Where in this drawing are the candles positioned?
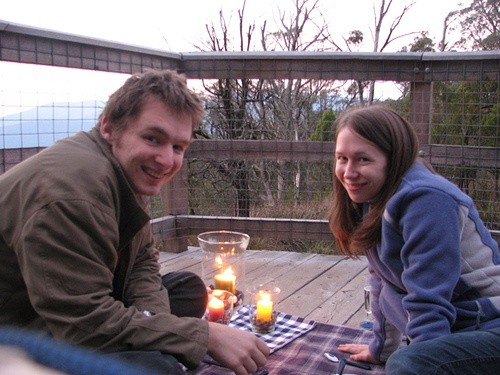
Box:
[214,265,237,295]
[255,291,274,322]
[206,296,228,323]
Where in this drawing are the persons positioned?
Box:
[328,105,500,375]
[0,68,271,375]
